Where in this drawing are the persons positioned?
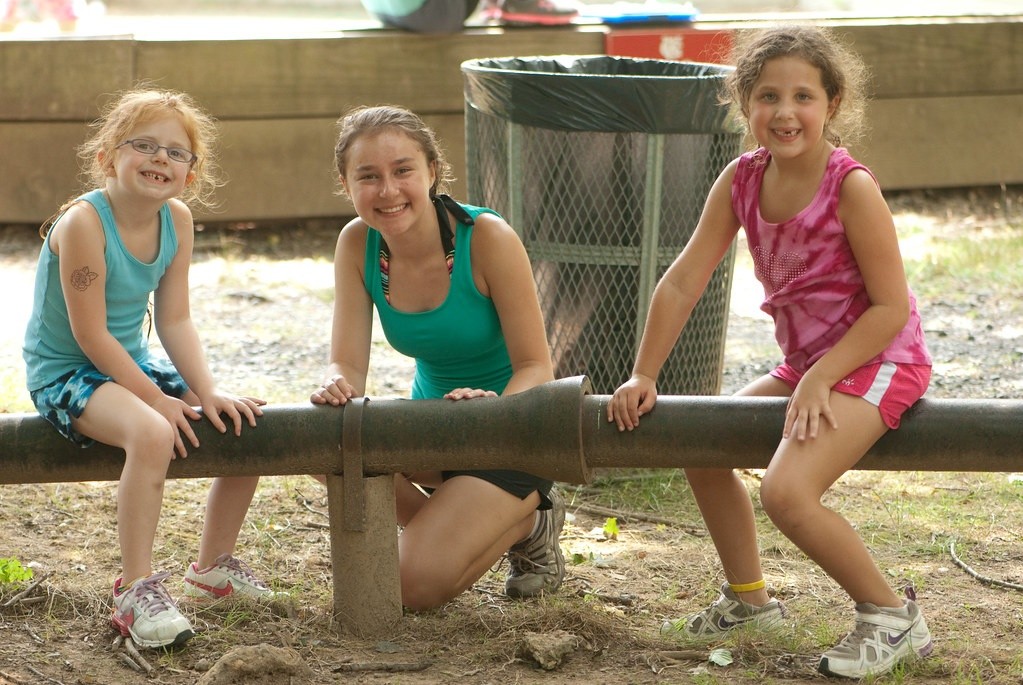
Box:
[605,22,937,682]
[306,103,570,614]
[20,84,293,653]
[352,0,585,39]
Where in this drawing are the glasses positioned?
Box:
[115,138,198,168]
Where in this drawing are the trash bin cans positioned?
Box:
[459,55,748,489]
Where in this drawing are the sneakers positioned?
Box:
[818,586,935,681]
[505,487,566,597]
[184,561,290,603]
[660,581,791,639]
[111,577,196,650]
